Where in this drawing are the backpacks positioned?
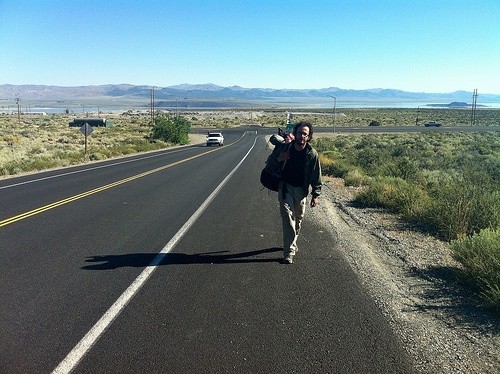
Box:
[260,140,292,193]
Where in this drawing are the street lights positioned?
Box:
[175,97,179,121]
[326,95,336,133]
[152,85,158,124]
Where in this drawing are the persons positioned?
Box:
[267,120,323,264]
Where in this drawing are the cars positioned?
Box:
[424,121,442,127]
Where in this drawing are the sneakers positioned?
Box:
[283,255,294,264]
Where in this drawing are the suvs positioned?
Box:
[206,133,225,147]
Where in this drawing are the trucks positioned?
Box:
[69,117,106,127]
